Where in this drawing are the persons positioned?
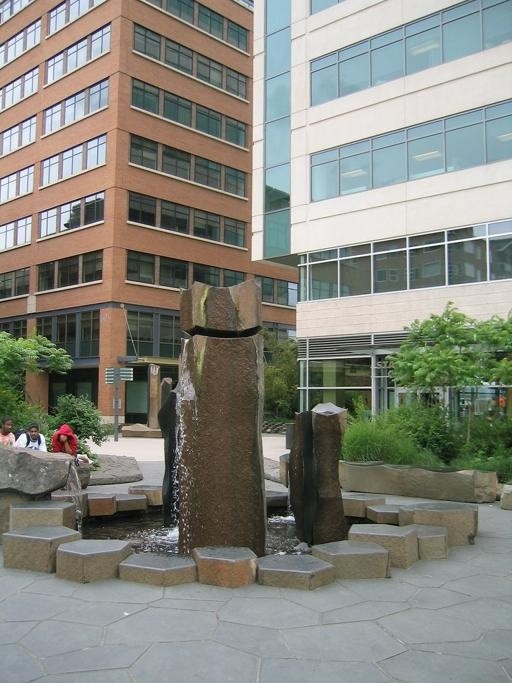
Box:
[51,422,77,455]
[15,421,47,453]
[0,415,17,450]
